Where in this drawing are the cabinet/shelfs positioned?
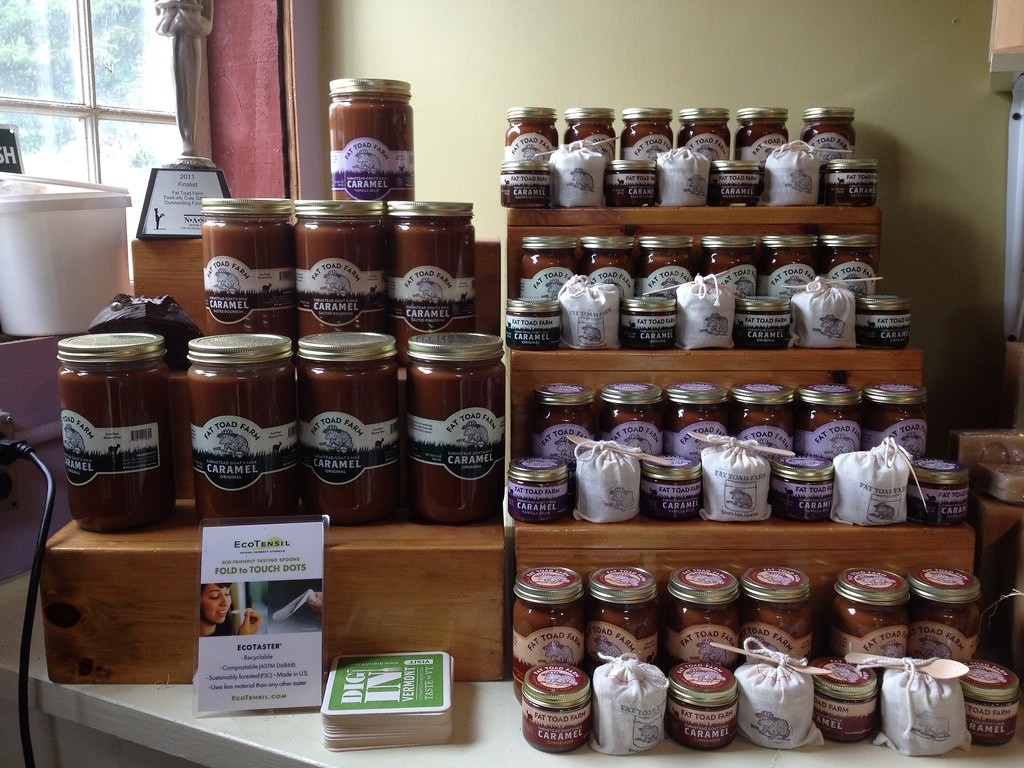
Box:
[41,237,509,681]
[501,202,980,605]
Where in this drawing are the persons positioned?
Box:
[306,591,322,622]
[200,582,262,636]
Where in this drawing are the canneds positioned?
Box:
[54,78,1022,752]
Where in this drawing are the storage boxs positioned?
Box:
[0,170,133,336]
